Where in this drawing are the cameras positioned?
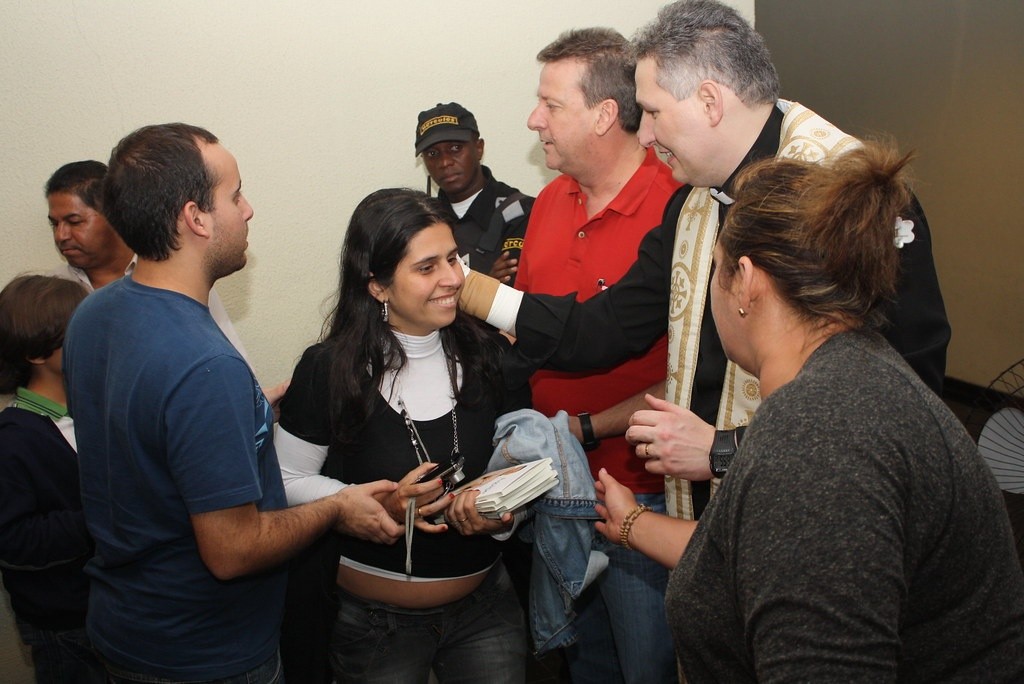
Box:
[413,452,467,488]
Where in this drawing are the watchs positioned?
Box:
[708,429,737,479]
[578,412,600,452]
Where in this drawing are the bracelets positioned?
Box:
[619,504,653,550]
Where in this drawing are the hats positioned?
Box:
[415,102,480,159]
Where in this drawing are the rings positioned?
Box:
[645,444,651,457]
[418,508,423,518]
[459,517,468,523]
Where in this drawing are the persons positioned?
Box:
[274,188,535,684]
[46,160,252,371]
[457,465,526,491]
[449,0,951,578]
[0,274,93,684]
[594,138,1024,684]
[64,123,405,684]
[492,26,684,684]
[414,102,539,410]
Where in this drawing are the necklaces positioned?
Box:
[389,360,464,490]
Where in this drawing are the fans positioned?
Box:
[964,358,1023,572]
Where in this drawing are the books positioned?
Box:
[434,458,560,525]
